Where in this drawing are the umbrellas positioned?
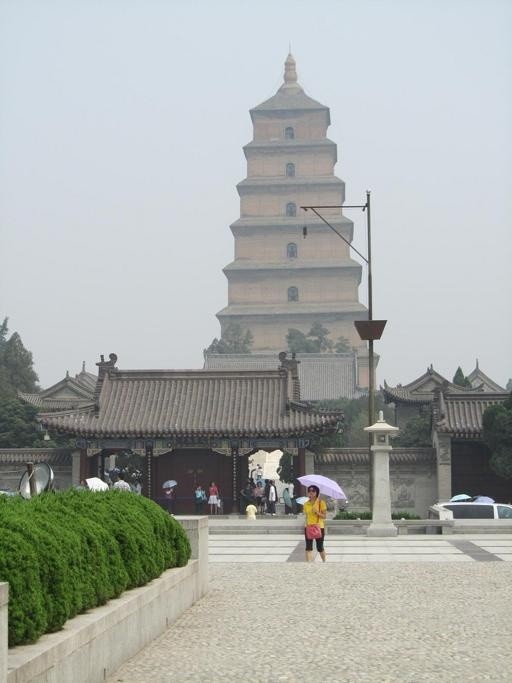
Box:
[450,492,472,500]
[295,495,311,505]
[295,473,346,499]
[473,496,494,504]
[162,479,179,488]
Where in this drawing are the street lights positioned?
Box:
[301,189,382,517]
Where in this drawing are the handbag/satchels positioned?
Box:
[305,523,323,540]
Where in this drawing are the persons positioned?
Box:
[302,484,327,561]
[195,484,207,514]
[73,464,143,499]
[239,464,298,516]
[207,480,219,514]
[166,487,177,514]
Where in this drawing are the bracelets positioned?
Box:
[314,511,318,514]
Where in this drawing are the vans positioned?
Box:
[429,499,512,519]
